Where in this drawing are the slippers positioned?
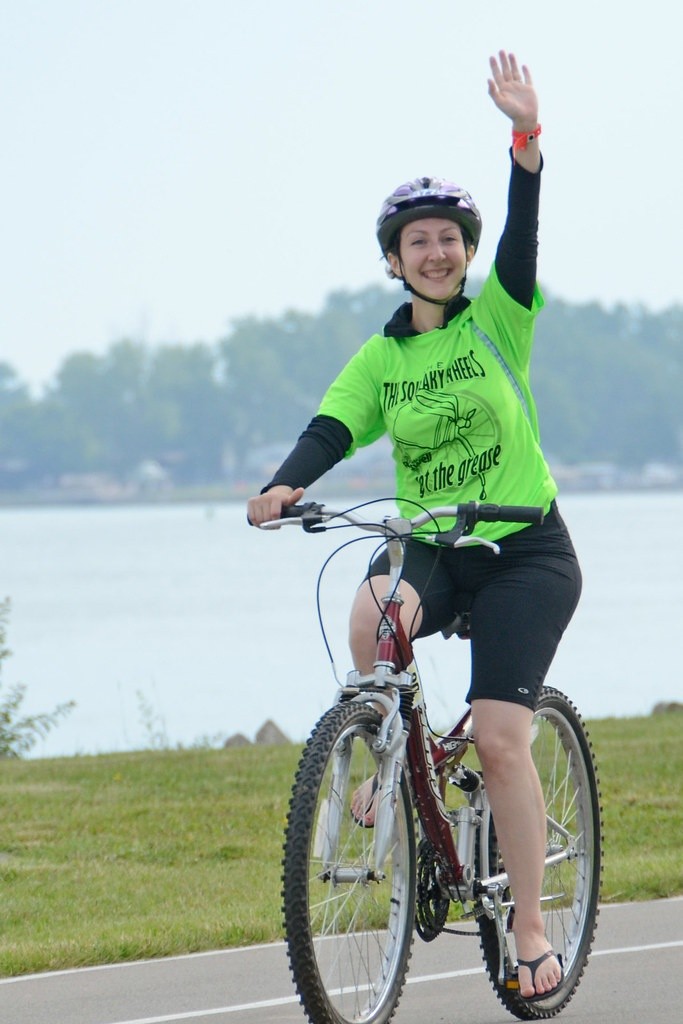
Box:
[350,772,383,829]
[516,948,564,1003]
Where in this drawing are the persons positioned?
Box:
[248,51,582,1002]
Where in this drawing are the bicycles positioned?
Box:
[248,501,605,1023]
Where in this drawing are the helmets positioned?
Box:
[375,176,483,262]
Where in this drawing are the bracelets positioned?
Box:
[512,124,542,166]
